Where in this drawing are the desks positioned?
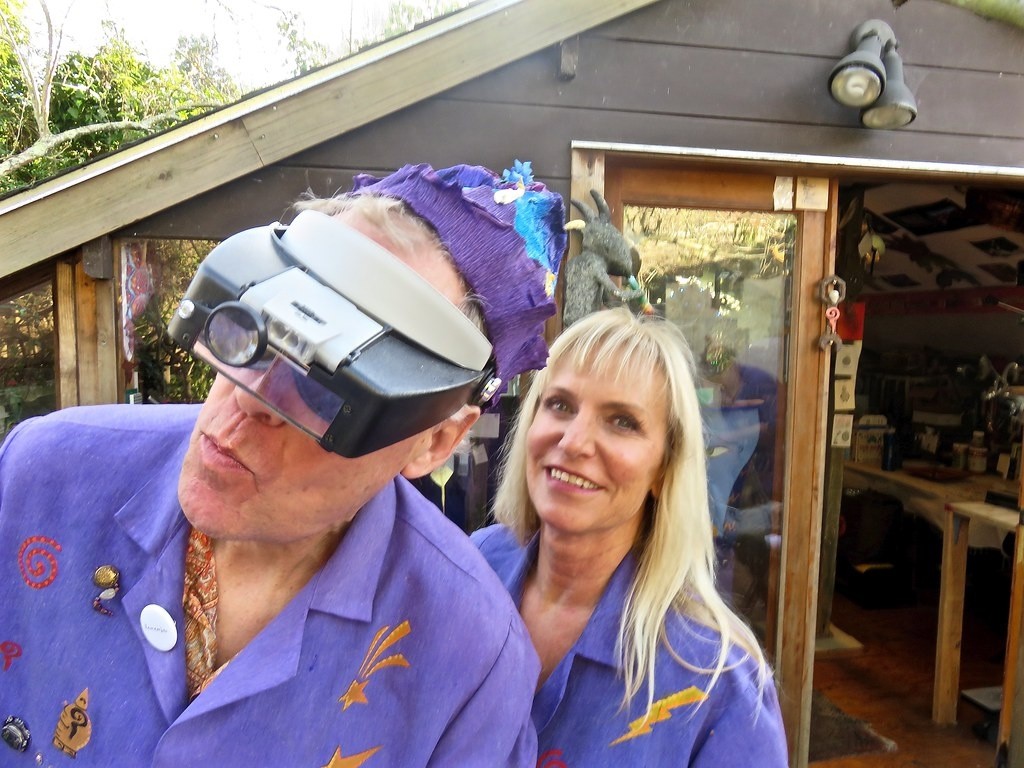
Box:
[844,458,1008,529]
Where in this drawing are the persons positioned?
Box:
[701,349,778,606]
[0,159,570,768]
[469,308,788,768]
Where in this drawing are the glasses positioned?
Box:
[166,207,504,459]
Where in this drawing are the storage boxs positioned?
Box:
[850,423,895,464]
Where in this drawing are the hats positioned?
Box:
[348,159,570,415]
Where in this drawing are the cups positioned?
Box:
[881,432,902,472]
[951,442,968,470]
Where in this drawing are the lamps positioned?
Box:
[825,18,919,130]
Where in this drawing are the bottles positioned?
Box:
[967,431,986,473]
